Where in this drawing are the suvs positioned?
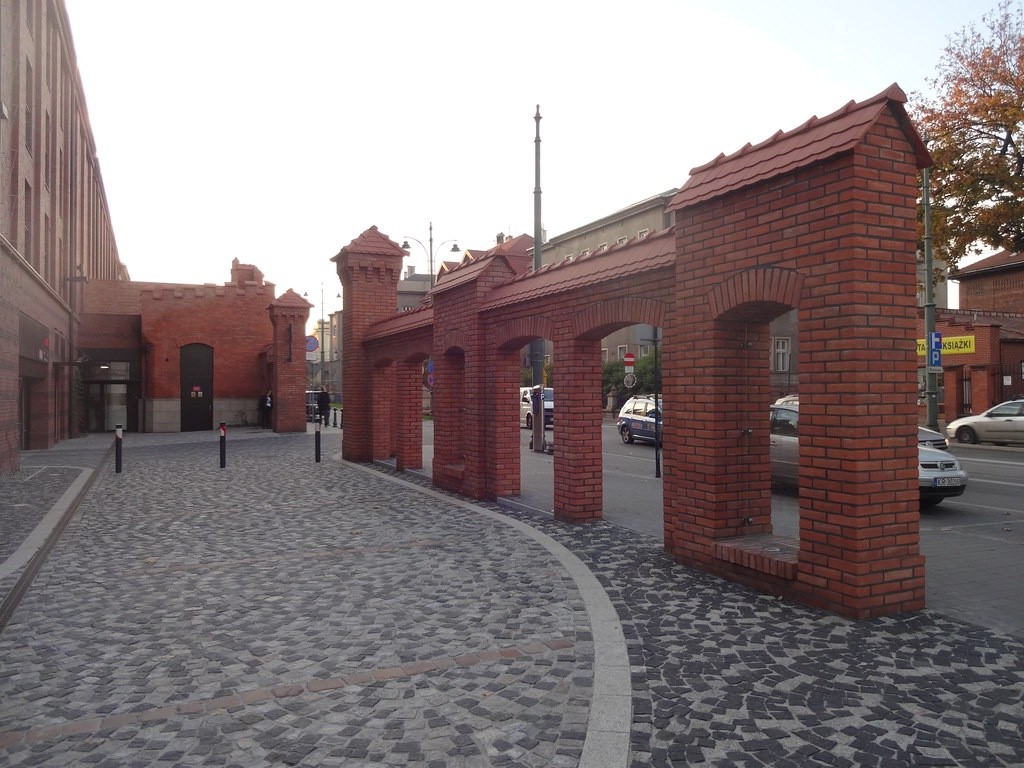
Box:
[305,390,325,420]
[775,392,952,454]
[615,394,663,447]
[944,394,1024,447]
[770,403,967,507]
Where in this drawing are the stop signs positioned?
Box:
[623,353,635,367]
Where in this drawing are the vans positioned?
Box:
[519,386,555,429]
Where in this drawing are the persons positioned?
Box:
[317,387,331,428]
[259,389,273,429]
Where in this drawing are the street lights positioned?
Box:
[400,221,462,290]
[302,282,341,390]
